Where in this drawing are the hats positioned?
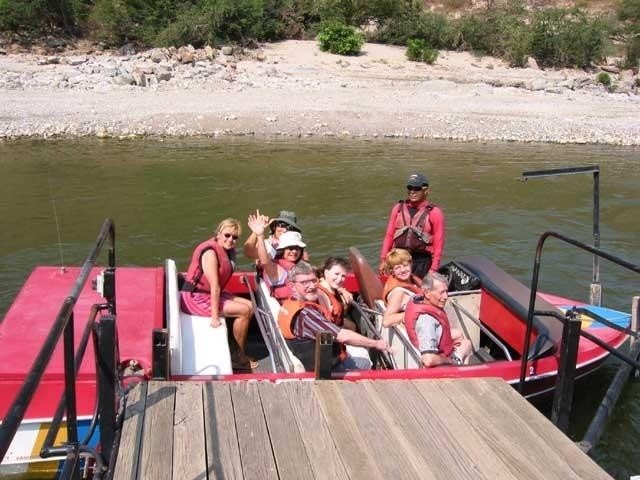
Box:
[269,210,302,233]
[406,173,429,187]
[276,231,306,249]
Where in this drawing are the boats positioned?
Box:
[0,258,632,421]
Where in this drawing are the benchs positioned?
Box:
[376,300,424,369]
[164,250,308,373]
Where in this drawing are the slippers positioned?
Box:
[232,351,258,370]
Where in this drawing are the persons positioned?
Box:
[404,272,463,367]
[316,256,356,332]
[379,173,444,275]
[244,208,309,276]
[247,213,310,306]
[382,248,472,365]
[180,218,258,367]
[277,262,392,372]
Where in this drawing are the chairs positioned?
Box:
[347,246,384,310]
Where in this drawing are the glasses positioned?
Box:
[407,185,427,191]
[294,279,319,285]
[224,233,238,240]
